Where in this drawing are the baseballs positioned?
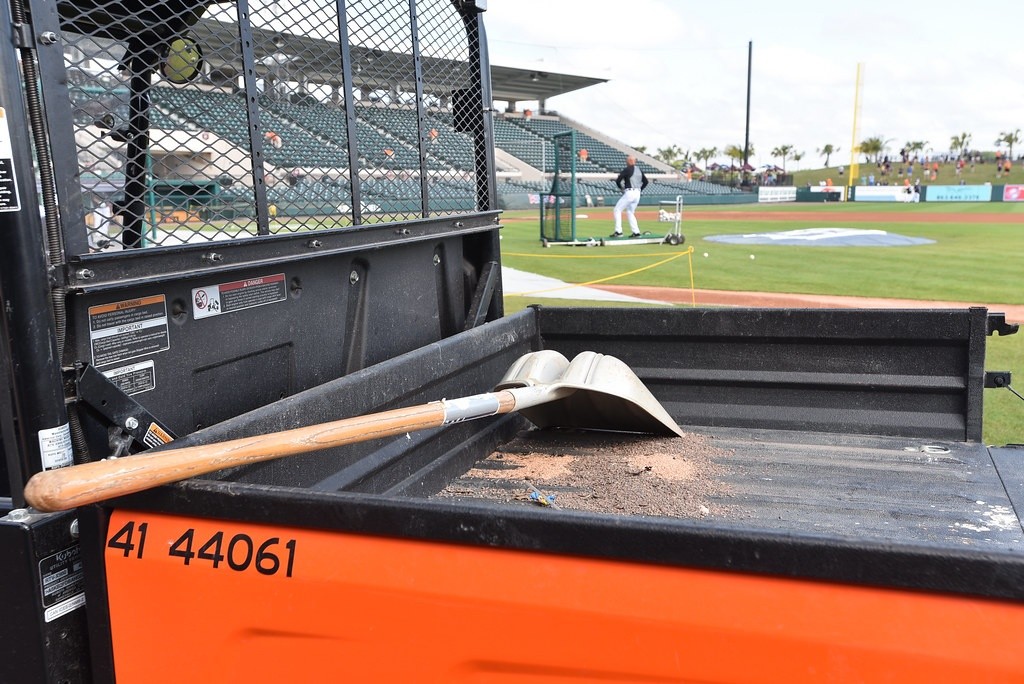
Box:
[703,252,709,257]
[750,255,755,260]
[500,235,503,239]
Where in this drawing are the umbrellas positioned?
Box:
[683,163,785,178]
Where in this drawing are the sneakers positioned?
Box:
[610,232,623,237]
[629,233,641,239]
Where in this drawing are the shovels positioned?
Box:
[22,349,684,512]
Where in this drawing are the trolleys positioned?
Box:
[663,195,685,245]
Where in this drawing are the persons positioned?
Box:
[383,149,395,161]
[819,178,834,186]
[525,110,532,122]
[860,149,939,185]
[580,148,588,163]
[838,166,844,178]
[609,155,648,238]
[428,128,438,144]
[939,150,955,165]
[995,150,1012,178]
[955,148,984,185]
[264,132,281,148]
[687,168,692,182]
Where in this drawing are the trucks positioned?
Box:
[0,0,1024,684]
